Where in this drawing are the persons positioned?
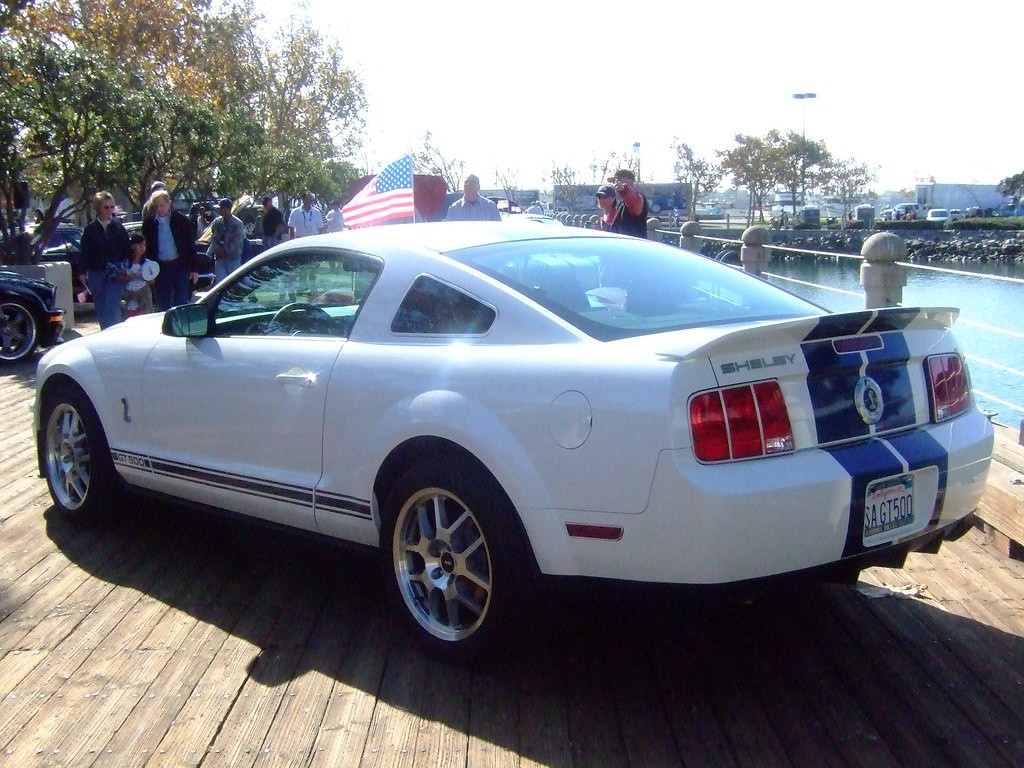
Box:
[251,191,343,305]
[526,201,545,215]
[208,198,245,286]
[777,209,789,229]
[847,211,854,220]
[591,169,648,299]
[673,207,681,230]
[78,181,219,331]
[891,207,917,220]
[446,174,502,221]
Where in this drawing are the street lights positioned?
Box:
[792,93,817,207]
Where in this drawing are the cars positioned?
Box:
[41,221,216,303]
[0,224,84,253]
[926,209,948,221]
[0,271,67,366]
[35,221,993,663]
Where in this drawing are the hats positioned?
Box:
[594,186,612,197]
[261,197,271,202]
[607,169,634,182]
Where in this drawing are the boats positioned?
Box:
[772,192,863,218]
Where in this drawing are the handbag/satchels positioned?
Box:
[206,244,220,259]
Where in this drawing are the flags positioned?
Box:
[341,155,416,231]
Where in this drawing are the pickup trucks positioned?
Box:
[884,203,928,218]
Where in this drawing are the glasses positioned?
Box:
[262,203,267,205]
[104,205,114,209]
[598,195,607,199]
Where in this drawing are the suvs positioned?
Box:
[238,205,265,245]
